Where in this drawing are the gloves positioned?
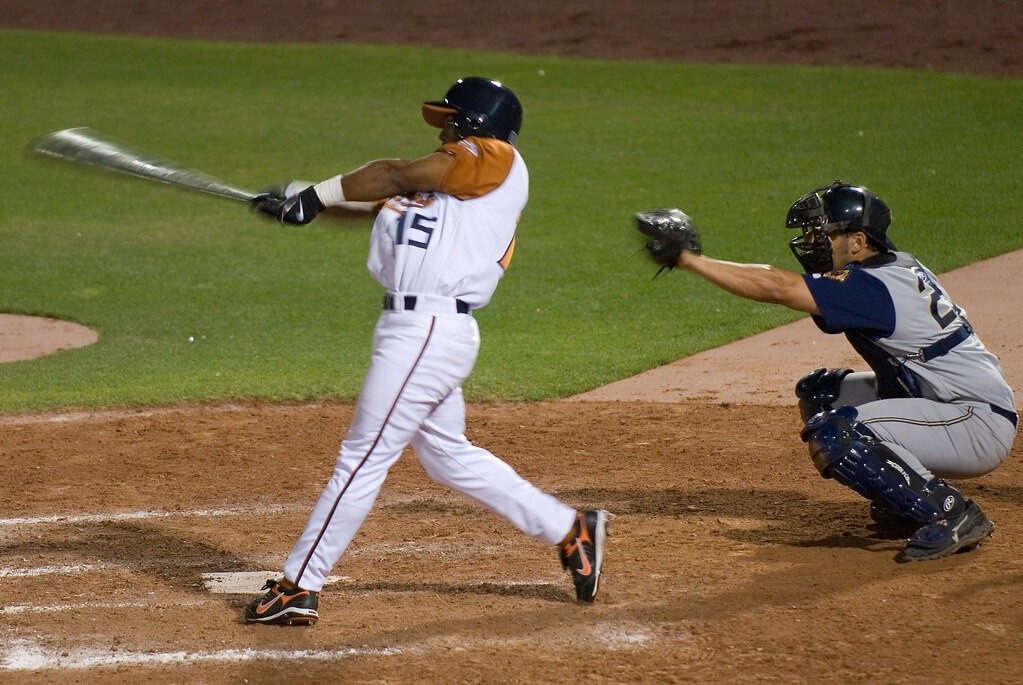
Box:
[251,185,327,225]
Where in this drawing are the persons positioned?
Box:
[635,179,1020,560]
[244,76,607,627]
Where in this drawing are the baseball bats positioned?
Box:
[28,125,268,203]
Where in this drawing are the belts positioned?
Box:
[381,294,469,315]
[990,403,1018,429]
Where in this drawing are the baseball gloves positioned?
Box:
[629,208,703,270]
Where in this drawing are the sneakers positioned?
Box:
[247,580,320,626]
[900,499,994,563]
[559,509,605,603]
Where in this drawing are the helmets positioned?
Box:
[824,185,898,252]
[421,77,522,145]
